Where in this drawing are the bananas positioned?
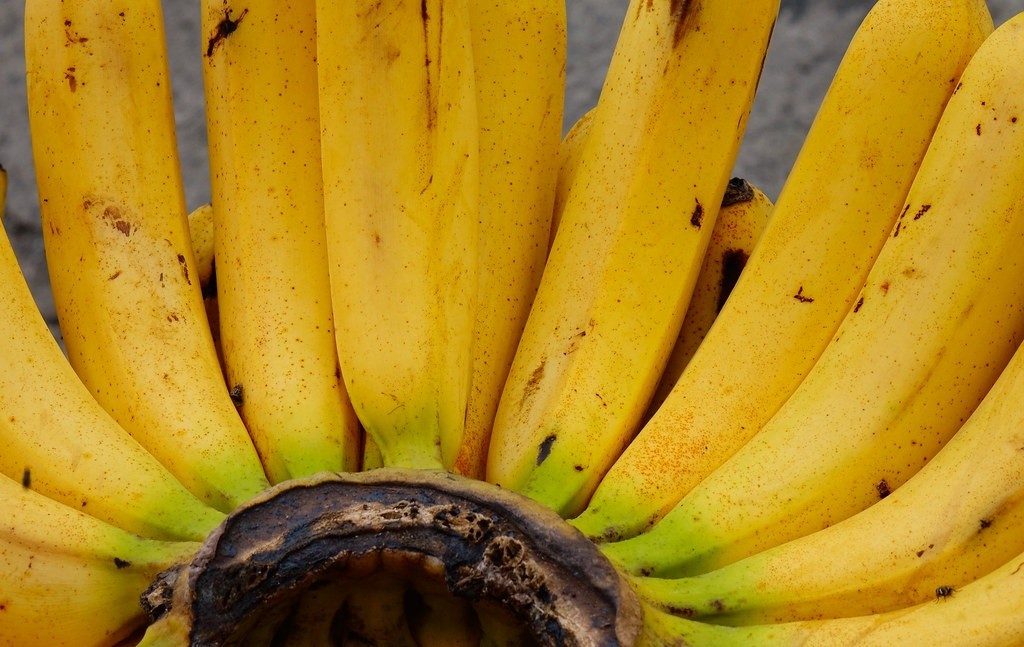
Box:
[0,0,1024,647]
